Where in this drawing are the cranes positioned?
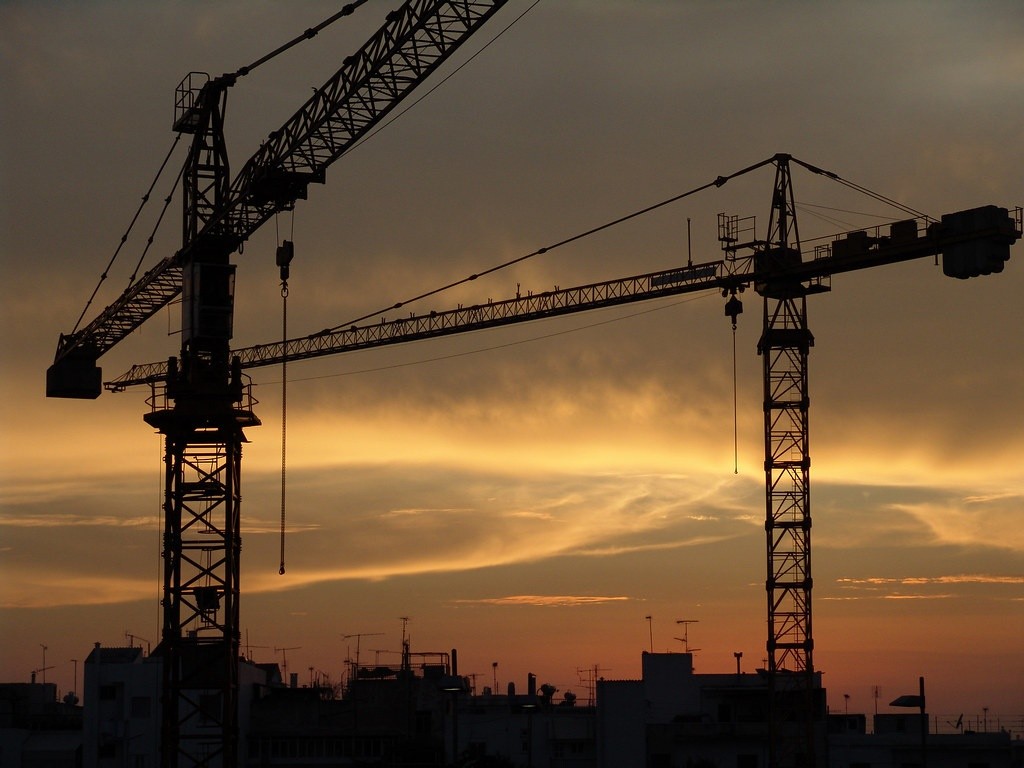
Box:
[45,2,541,650]
[103,153,1024,675]
